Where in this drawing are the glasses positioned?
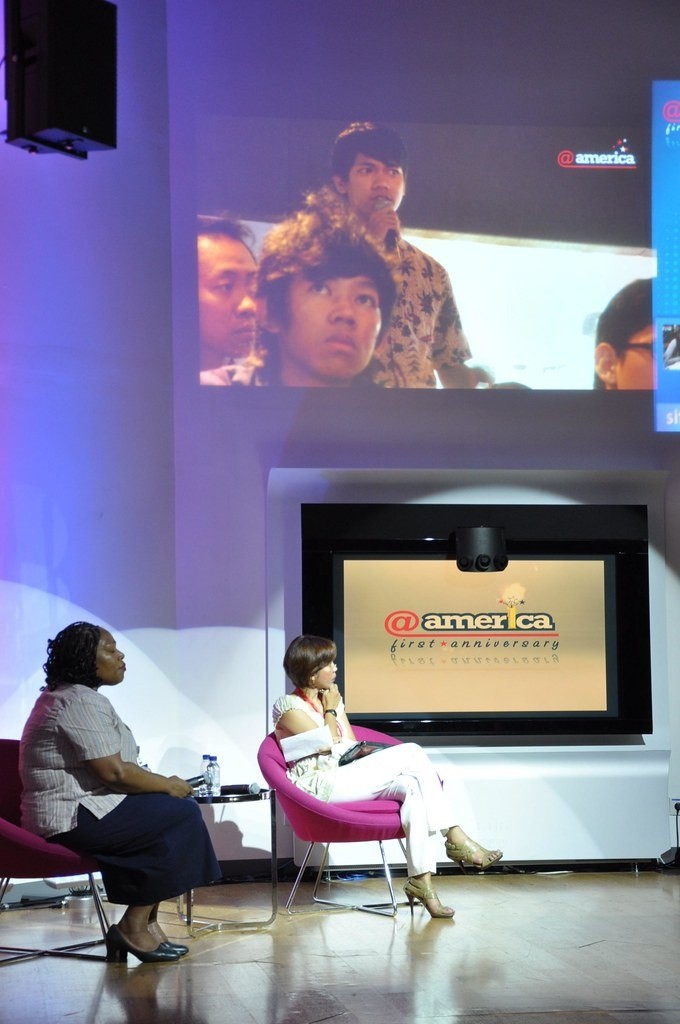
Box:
[613,341,653,357]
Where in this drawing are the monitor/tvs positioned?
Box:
[302,538,654,736]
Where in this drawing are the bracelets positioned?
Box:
[325,710,337,717]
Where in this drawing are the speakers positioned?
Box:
[5,0,118,156]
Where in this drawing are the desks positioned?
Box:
[176,787,278,940]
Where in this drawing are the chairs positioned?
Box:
[0,739,111,965]
[257,724,444,918]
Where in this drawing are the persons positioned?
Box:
[199,203,399,386]
[332,123,493,389]
[18,622,220,960]
[198,215,260,370]
[272,635,503,918]
[595,280,654,390]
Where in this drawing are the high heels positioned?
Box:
[403,876,454,918]
[106,923,180,962]
[445,839,503,875]
[159,942,188,956]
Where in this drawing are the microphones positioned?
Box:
[373,198,397,251]
[220,783,261,795]
[186,774,211,788]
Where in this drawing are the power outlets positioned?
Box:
[669,795,680,816]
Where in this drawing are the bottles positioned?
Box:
[207,756,221,796]
[199,754,211,796]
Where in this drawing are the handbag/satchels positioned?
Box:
[337,740,396,766]
